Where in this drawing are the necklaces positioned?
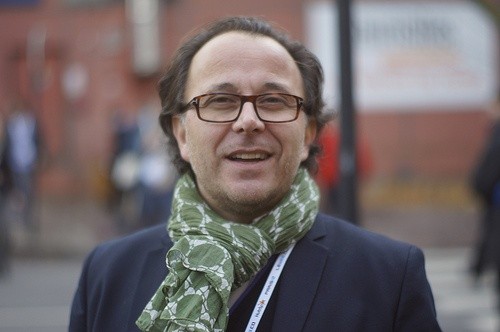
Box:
[236,239,297,331]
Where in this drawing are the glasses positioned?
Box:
[177,90,314,124]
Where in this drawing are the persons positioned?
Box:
[98,83,376,224]
[70,16,445,332]
[466,124,500,287]
[3,99,48,247]
